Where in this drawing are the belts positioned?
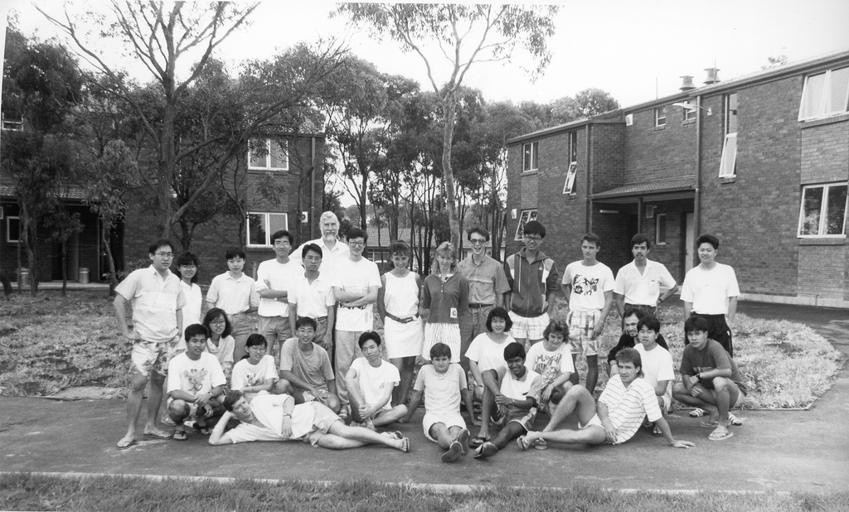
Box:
[468,303,493,308]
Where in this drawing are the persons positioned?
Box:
[680,235,744,426]
[107,209,695,466]
[673,317,748,440]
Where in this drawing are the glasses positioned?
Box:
[471,239,487,244]
[155,252,173,257]
[209,319,225,324]
[526,236,541,240]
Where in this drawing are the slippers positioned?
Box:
[384,429,548,462]
[645,407,743,441]
[117,420,213,449]
